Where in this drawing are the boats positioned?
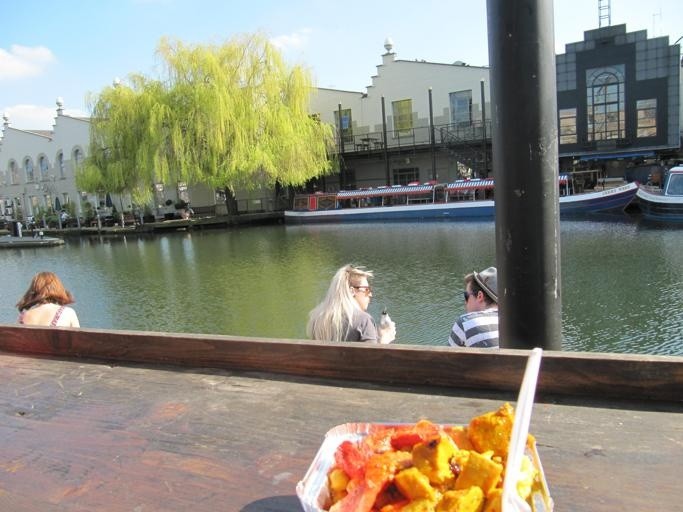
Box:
[0,236,65,247]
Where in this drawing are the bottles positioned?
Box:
[380,311,392,331]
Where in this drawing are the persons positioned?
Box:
[173,202,195,220]
[60,210,69,229]
[13,270,80,329]
[445,266,500,349]
[303,263,398,346]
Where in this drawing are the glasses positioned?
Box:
[353,286,371,297]
[462,291,475,303]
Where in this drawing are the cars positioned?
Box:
[0,208,155,229]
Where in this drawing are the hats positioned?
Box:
[472,267,498,304]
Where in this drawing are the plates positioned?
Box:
[296,422,554,512]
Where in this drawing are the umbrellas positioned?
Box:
[104,191,113,214]
[54,197,62,229]
